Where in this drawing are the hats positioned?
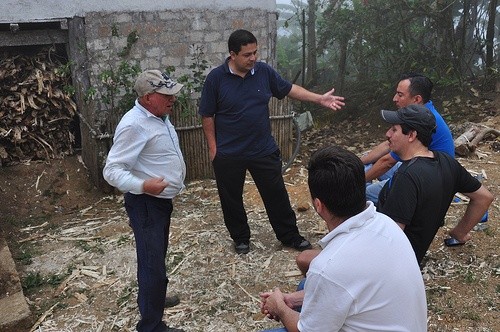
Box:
[134,69,184,97]
[380,104,437,135]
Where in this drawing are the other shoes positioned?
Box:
[164,295,180,307]
[160,323,184,332]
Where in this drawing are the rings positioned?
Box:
[334,103,336,106]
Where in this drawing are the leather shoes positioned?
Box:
[281,236,312,251]
[234,242,250,254]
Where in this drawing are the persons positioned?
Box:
[198,29,346,254]
[261,144,427,332]
[297,104,494,277]
[102,70,187,332]
[358,75,456,207]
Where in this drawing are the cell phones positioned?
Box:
[444,238,466,247]
